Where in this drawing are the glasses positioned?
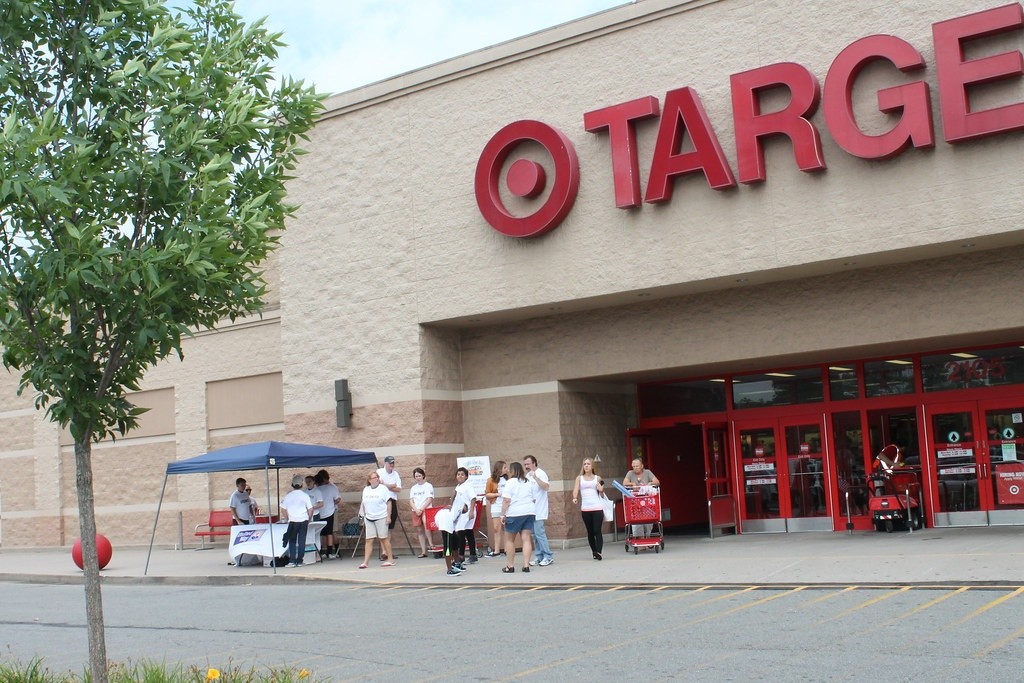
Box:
[369,476,380,480]
[245,489,252,492]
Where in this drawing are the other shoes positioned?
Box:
[596,552,602,561]
[285,563,296,567]
[382,553,388,559]
[447,551,506,576]
[328,554,335,559]
[320,553,326,558]
[297,562,306,567]
[539,558,553,566]
[593,555,598,559]
[529,558,542,566]
[392,555,398,559]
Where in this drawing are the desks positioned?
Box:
[228,521,327,567]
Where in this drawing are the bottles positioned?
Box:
[249,513,254,525]
[281,516,285,523]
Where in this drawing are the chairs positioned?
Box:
[335,516,365,559]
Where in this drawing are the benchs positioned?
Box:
[194,511,233,551]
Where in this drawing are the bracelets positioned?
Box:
[573,497,578,499]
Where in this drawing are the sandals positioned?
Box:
[522,567,530,572]
[502,566,514,572]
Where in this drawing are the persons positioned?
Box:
[303,476,324,522]
[485,461,509,557]
[358,472,395,568]
[450,467,478,564]
[623,459,660,551]
[573,458,604,560]
[434,506,466,576]
[281,476,313,568]
[245,484,259,525]
[229,478,253,526]
[315,469,341,558]
[376,456,402,559]
[500,462,536,572]
[409,468,436,558]
[524,455,553,566]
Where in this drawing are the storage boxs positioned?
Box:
[288,545,317,565]
[263,556,273,568]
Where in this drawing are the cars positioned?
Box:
[747,452,1024,508]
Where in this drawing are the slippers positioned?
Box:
[381,562,396,566]
[418,554,428,558]
[359,563,368,568]
[427,546,436,550]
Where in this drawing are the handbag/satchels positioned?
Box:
[599,491,614,522]
[342,522,361,536]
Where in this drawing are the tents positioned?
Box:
[145,441,415,574]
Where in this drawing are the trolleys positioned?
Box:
[620,483,667,555]
[423,492,490,559]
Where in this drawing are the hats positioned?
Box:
[384,456,396,464]
[291,475,303,486]
